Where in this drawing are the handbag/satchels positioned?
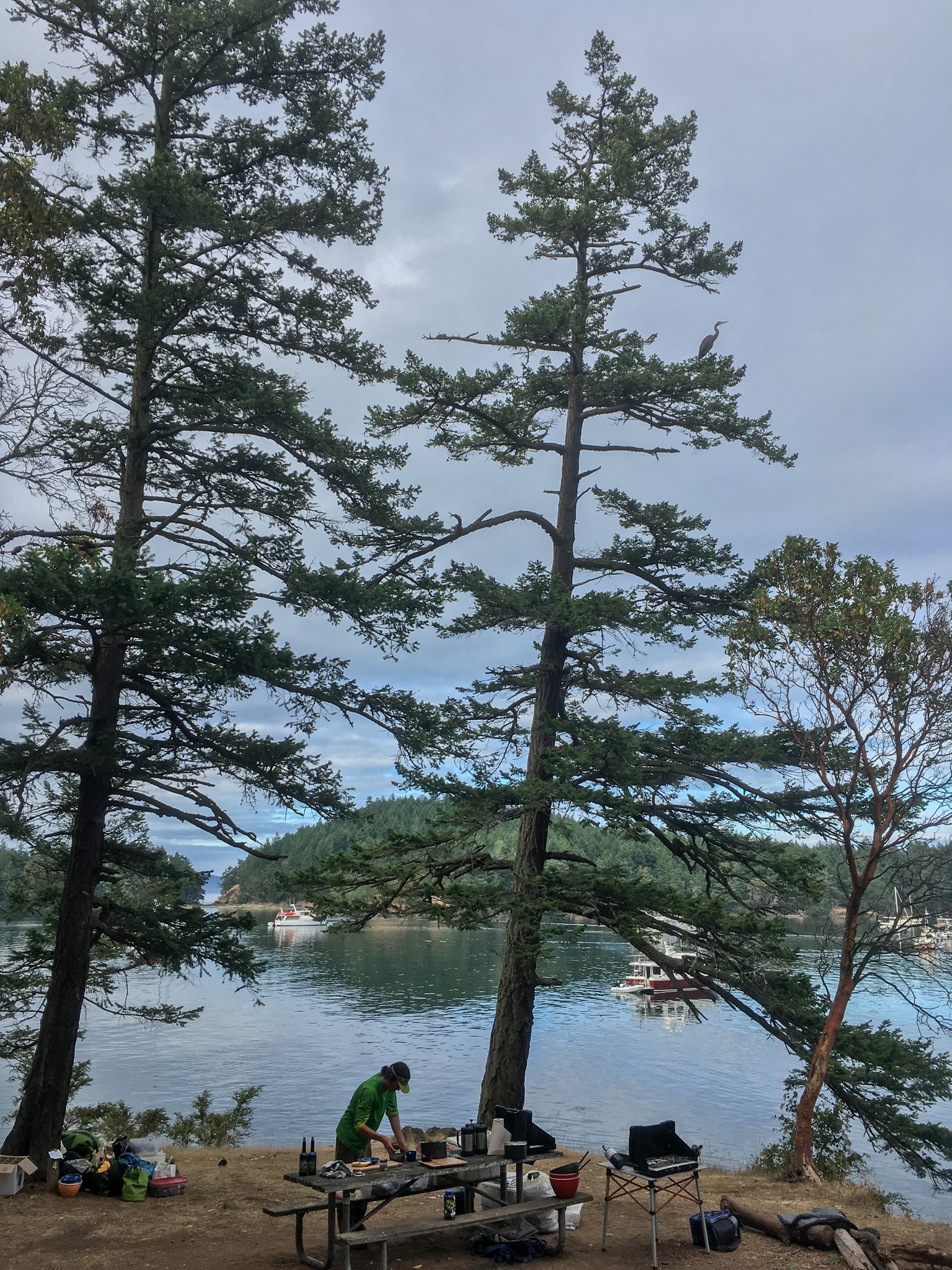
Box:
[106,1134,176,1202]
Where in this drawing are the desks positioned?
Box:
[596,1161,710,1270]
[283,1149,564,1270]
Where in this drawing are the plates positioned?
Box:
[357,1157,379,1164]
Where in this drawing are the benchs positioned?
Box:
[263,1176,594,1270]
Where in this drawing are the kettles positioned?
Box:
[467,1119,478,1154]
[475,1121,488,1155]
[457,1124,474,1157]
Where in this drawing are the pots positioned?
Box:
[504,1140,527,1160]
[420,1141,447,1159]
[550,1151,591,1174]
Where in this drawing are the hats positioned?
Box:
[390,1061,411,1094]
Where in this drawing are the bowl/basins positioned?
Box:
[389,1154,405,1162]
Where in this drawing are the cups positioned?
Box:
[406,1151,416,1162]
[421,1153,432,1162]
[549,1172,581,1198]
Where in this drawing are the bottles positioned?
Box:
[443,1191,455,1220]
[308,1136,316,1175]
[299,1137,308,1176]
[602,1145,624,1169]
[380,1159,388,1171]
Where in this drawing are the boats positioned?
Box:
[626,941,716,992]
[610,980,644,994]
[274,897,329,926]
[876,887,952,951]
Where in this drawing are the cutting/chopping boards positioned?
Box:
[345,1160,402,1172]
[417,1156,468,1168]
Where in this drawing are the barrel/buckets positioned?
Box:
[59,1179,82,1197]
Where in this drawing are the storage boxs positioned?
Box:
[479,1171,517,1208]
[130,1138,161,1157]
[0,1155,38,1195]
[149,1177,189,1198]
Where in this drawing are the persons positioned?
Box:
[335,1061,411,1251]
